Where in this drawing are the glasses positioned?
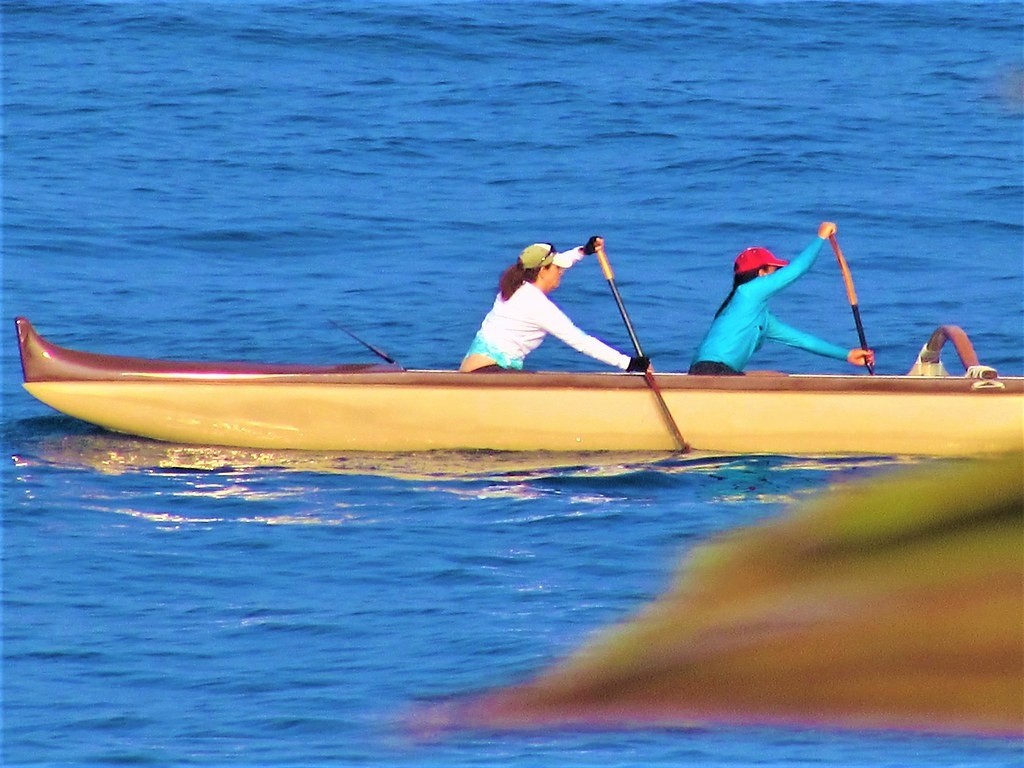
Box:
[539,242,555,267]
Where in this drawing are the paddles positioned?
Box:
[330,319,400,364]
[828,233,875,376]
[594,243,692,454]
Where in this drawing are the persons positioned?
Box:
[688,221,875,376]
[460,236,655,374]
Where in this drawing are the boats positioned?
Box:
[13,316,1024,461]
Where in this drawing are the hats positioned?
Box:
[519,243,573,272]
[735,247,788,275]
